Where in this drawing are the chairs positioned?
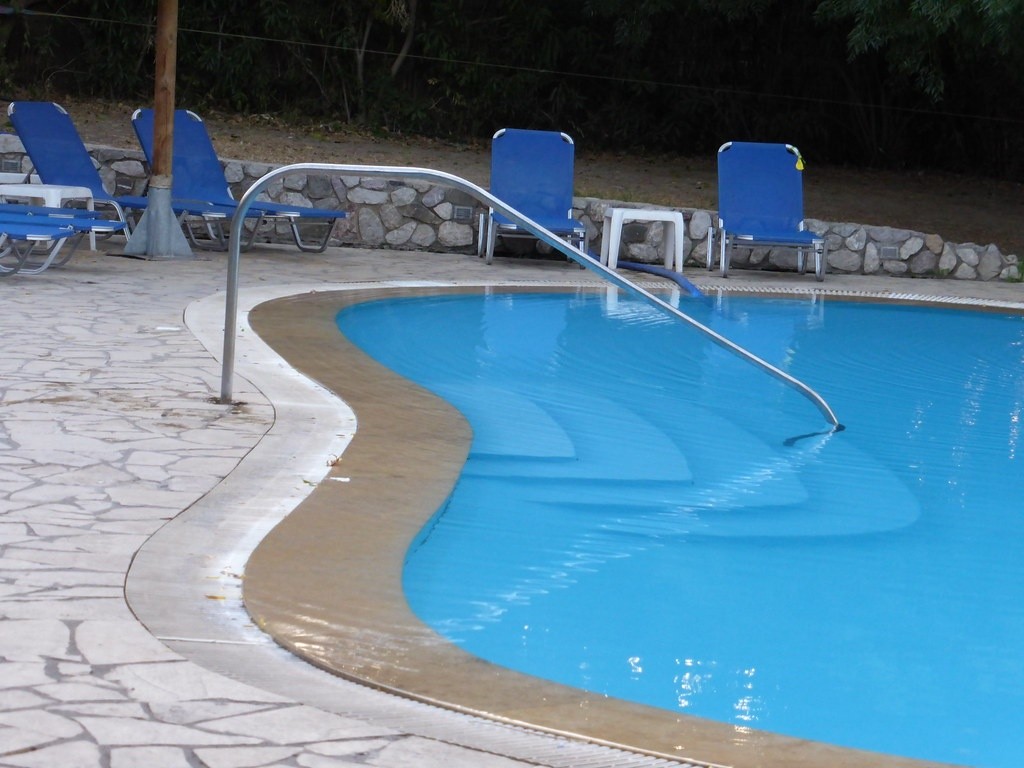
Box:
[0,223,73,277]
[706,140,828,281]
[7,102,265,252]
[0,213,126,268]
[131,108,346,253]
[0,203,102,255]
[476,129,587,270]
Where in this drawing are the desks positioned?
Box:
[0,184,96,251]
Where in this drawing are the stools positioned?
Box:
[600,208,683,273]
